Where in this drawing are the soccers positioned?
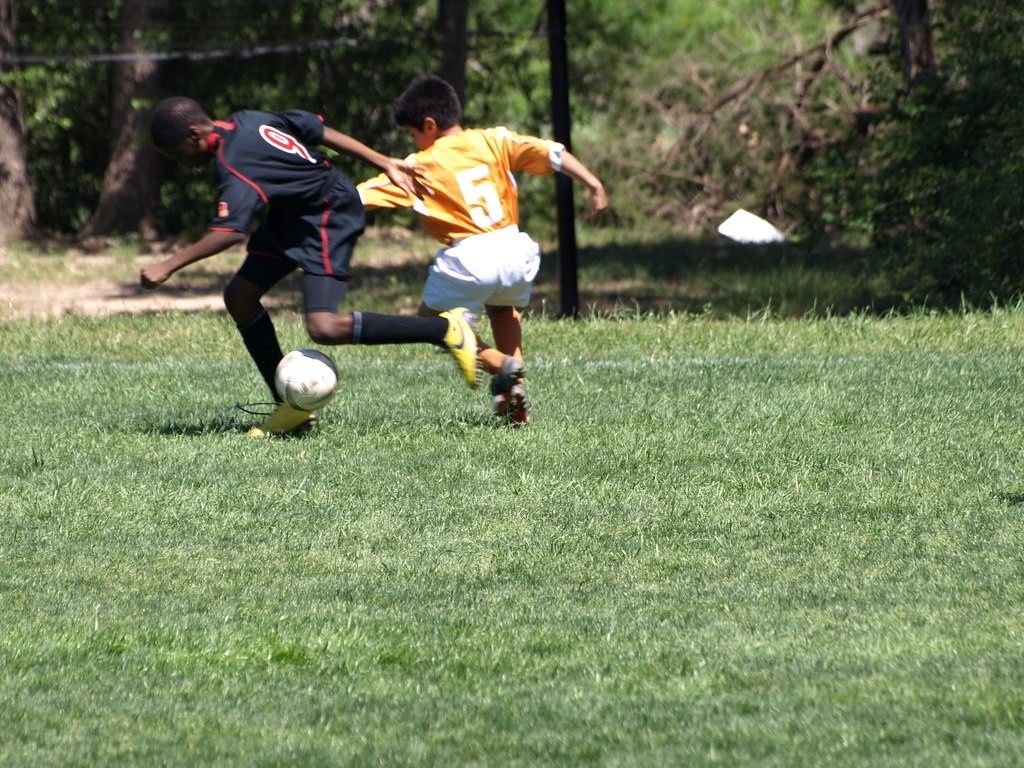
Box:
[274,348,339,411]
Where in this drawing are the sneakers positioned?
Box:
[438,307,483,389]
[235,402,318,438]
[489,357,526,396]
[494,387,529,428]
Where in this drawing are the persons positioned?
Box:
[352,75,608,432]
[137,94,489,439]
